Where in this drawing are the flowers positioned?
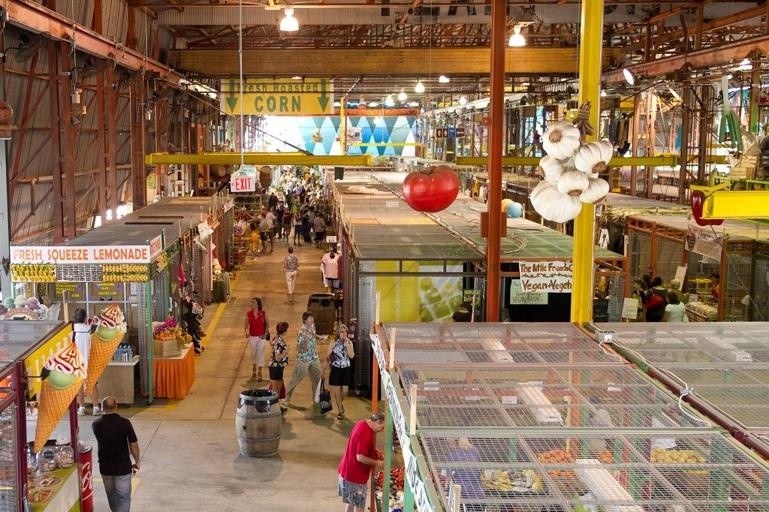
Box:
[154,317,188,349]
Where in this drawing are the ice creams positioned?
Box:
[34,342,84,452]
[85,304,128,397]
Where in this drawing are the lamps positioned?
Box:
[507,20,531,49]
[143,5,154,121]
[621,65,639,86]
[277,2,302,33]
[69,0,83,105]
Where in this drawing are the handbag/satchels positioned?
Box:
[269,381,285,399]
[320,390,333,413]
[330,351,341,364]
[266,326,270,340]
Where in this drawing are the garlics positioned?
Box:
[530,122,614,223]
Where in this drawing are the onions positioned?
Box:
[537,448,576,478]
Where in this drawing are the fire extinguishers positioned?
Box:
[348,318,357,340]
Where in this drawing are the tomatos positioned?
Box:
[402,165,459,213]
[378,467,445,490]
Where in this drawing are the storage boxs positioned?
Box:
[153,339,181,357]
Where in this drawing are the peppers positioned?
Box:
[691,168,726,226]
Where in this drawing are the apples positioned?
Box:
[650,447,697,465]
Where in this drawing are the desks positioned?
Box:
[25,465,83,512]
[70,355,139,407]
[139,342,197,402]
[213,277,226,302]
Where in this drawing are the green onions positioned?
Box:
[718,75,743,152]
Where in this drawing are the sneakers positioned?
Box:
[78,408,85,414]
[92,406,100,415]
[338,405,345,419]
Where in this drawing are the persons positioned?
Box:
[596,267,720,322]
[284,246,300,302]
[243,296,270,381]
[326,323,356,420]
[73,308,101,416]
[336,412,385,512]
[91,396,141,512]
[270,321,291,414]
[322,249,340,289]
[285,310,324,405]
[255,165,334,252]
